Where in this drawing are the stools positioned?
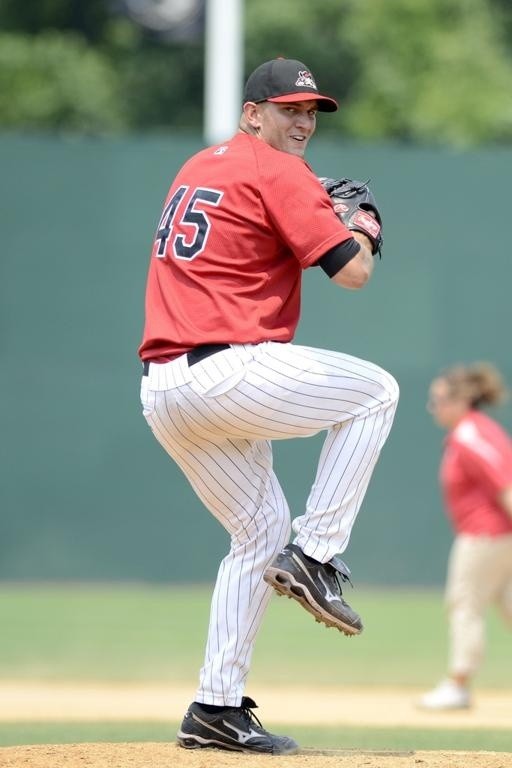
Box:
[142,343,231,377]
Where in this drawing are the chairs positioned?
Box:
[263,543,363,637]
[177,696,299,756]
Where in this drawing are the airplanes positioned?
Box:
[322,176,382,257]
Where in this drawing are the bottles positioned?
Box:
[243,57,338,113]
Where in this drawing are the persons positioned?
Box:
[417,364,512,712]
[138,58,400,756]
